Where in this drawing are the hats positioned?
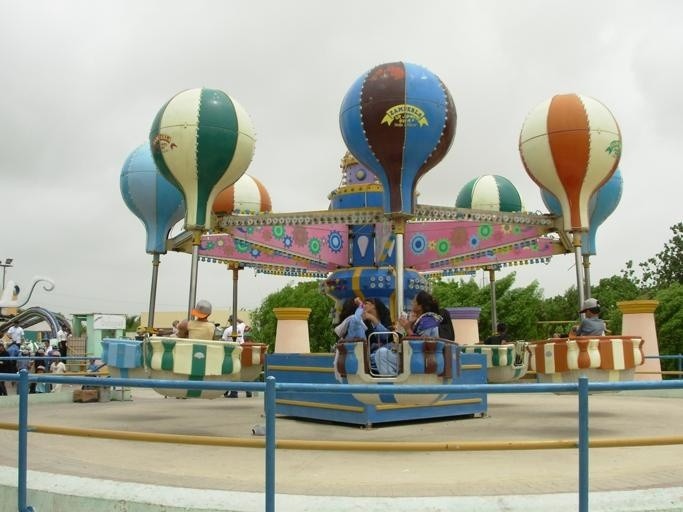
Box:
[192,300,213,320]
[578,298,600,314]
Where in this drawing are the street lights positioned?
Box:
[0,258,14,289]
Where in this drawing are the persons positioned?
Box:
[485,322,508,345]
[338,295,368,338]
[0,319,72,396]
[435,308,455,340]
[374,291,445,376]
[171,300,245,344]
[576,298,605,336]
[364,298,395,367]
